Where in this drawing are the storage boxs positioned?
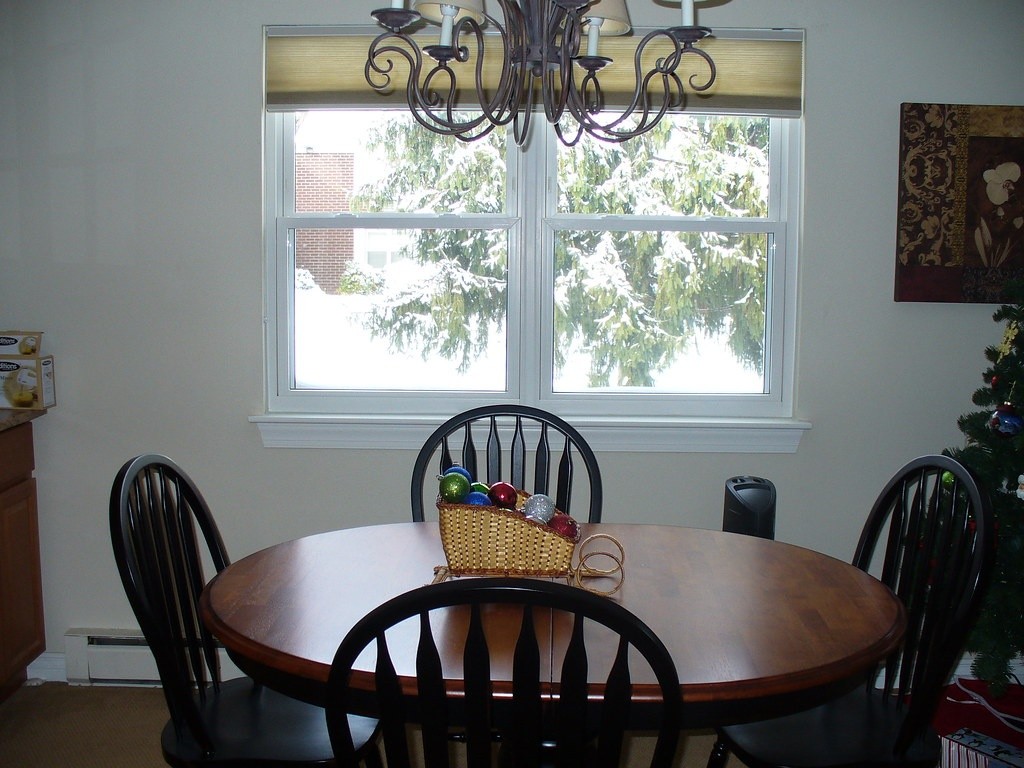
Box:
[0,330,57,411]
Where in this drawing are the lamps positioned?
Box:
[364,0,731,147]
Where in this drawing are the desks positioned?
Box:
[197,522,904,768]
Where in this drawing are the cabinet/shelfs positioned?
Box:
[0,422,46,702]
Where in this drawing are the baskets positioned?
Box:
[436,480,583,578]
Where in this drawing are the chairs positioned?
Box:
[707,454,996,768]
[109,454,382,768]
[325,578,684,768]
[411,404,603,523]
